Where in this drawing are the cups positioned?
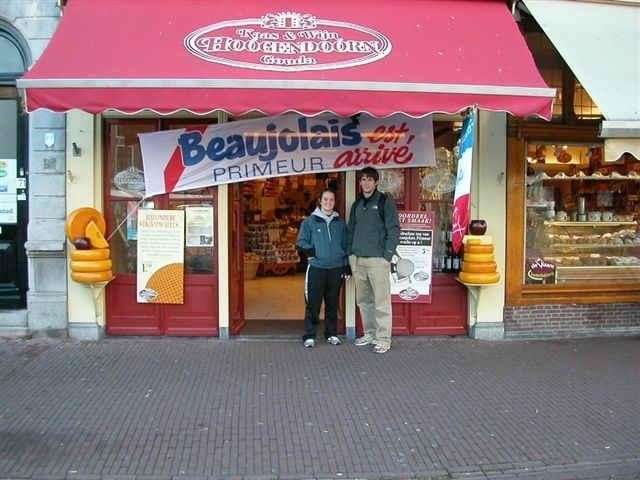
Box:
[602,212,613,222]
[577,214,587,222]
[588,211,601,221]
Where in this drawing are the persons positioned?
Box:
[346,166,401,354]
[294,188,348,348]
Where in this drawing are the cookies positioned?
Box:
[144,263,183,304]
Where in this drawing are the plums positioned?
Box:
[470,220,486,235]
[74,239,89,249]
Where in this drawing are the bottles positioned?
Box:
[577,197,585,215]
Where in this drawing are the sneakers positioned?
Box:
[303,338,315,348]
[373,343,391,353]
[327,336,342,345]
[354,336,377,346]
